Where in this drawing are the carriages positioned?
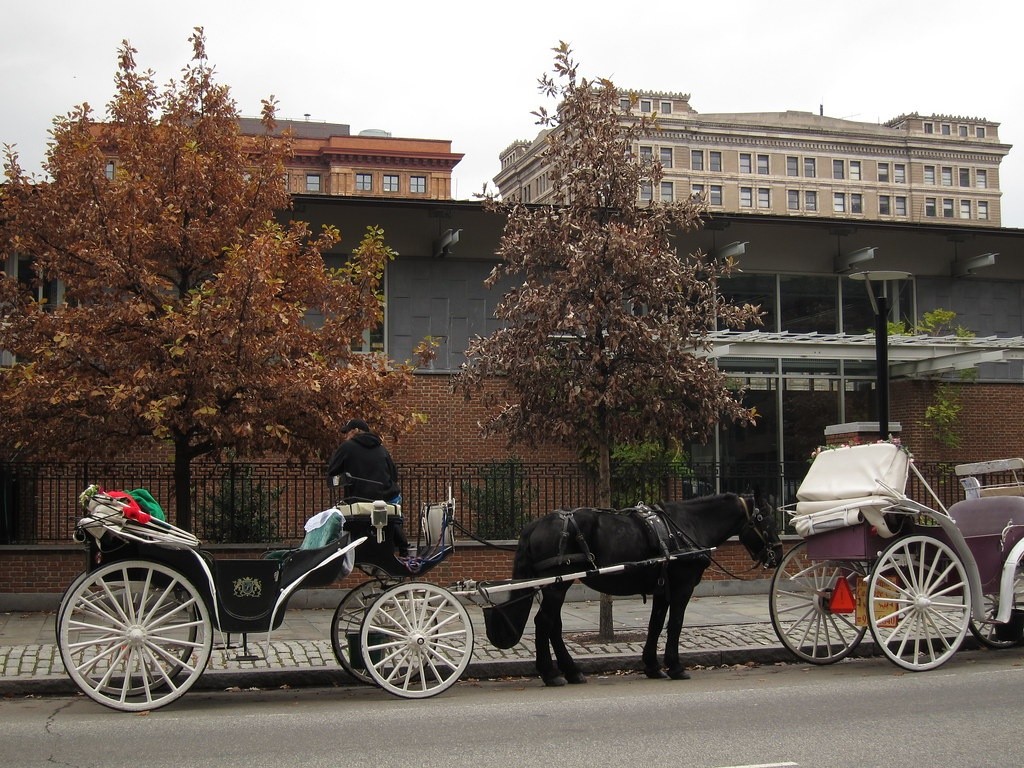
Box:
[768,440,1024,671]
[54,483,784,713]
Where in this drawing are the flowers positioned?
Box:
[805,434,915,465]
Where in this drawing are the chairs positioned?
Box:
[326,471,401,516]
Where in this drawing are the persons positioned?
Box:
[327,419,401,503]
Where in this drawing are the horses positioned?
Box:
[508,492,785,688]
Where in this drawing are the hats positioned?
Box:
[340,418,369,434]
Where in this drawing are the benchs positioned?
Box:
[264,508,340,559]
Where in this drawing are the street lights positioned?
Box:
[848,270,914,443]
